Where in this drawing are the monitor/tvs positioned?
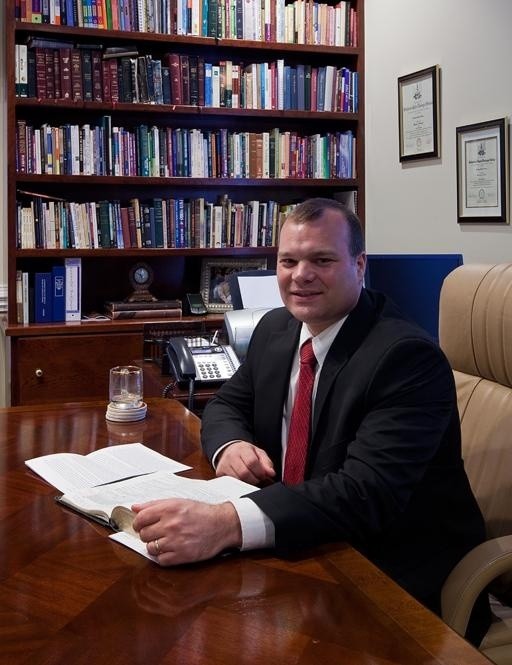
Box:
[362,255,462,342]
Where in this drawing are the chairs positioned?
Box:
[439,262,512,665]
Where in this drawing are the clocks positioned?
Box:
[126,262,157,303]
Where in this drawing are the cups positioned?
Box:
[108,431,144,447]
[109,365,143,409]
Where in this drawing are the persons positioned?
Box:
[132,199,504,647]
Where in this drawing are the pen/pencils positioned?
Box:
[212,331,218,343]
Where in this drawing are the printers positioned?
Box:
[224,270,287,362]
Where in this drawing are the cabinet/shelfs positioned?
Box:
[1,0,365,405]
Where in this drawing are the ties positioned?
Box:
[283,340,317,487]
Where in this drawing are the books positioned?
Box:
[17,116,357,178]
[101,295,182,320]
[11,0,358,46]
[15,255,81,324]
[55,470,264,545]
[16,194,360,252]
[15,51,358,111]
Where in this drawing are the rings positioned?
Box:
[154,539,163,554]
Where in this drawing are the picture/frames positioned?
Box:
[398,64,441,161]
[201,256,268,312]
[456,115,511,225]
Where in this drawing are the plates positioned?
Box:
[104,420,146,433]
[105,402,148,423]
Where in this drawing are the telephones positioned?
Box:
[164,336,241,389]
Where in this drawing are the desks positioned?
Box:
[134,358,216,417]
[0,397,495,665]
